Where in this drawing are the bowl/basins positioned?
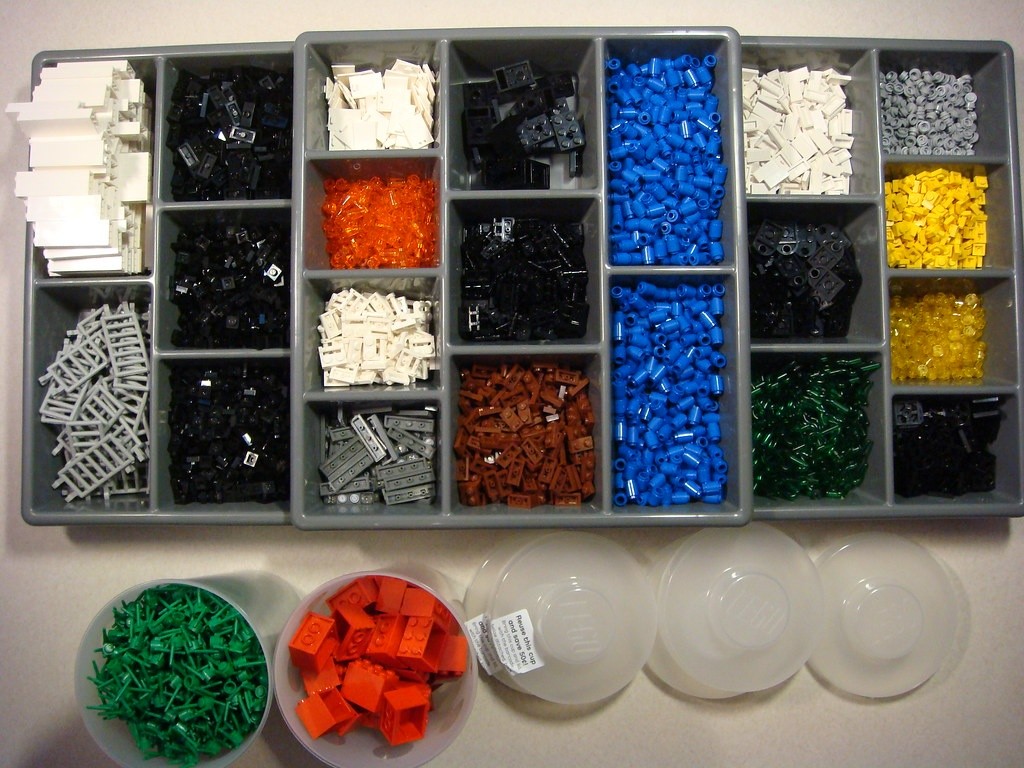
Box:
[273,565,478,768]
[463,523,958,705]
[73,579,301,768]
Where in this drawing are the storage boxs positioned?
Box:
[18,26,1024,525]
